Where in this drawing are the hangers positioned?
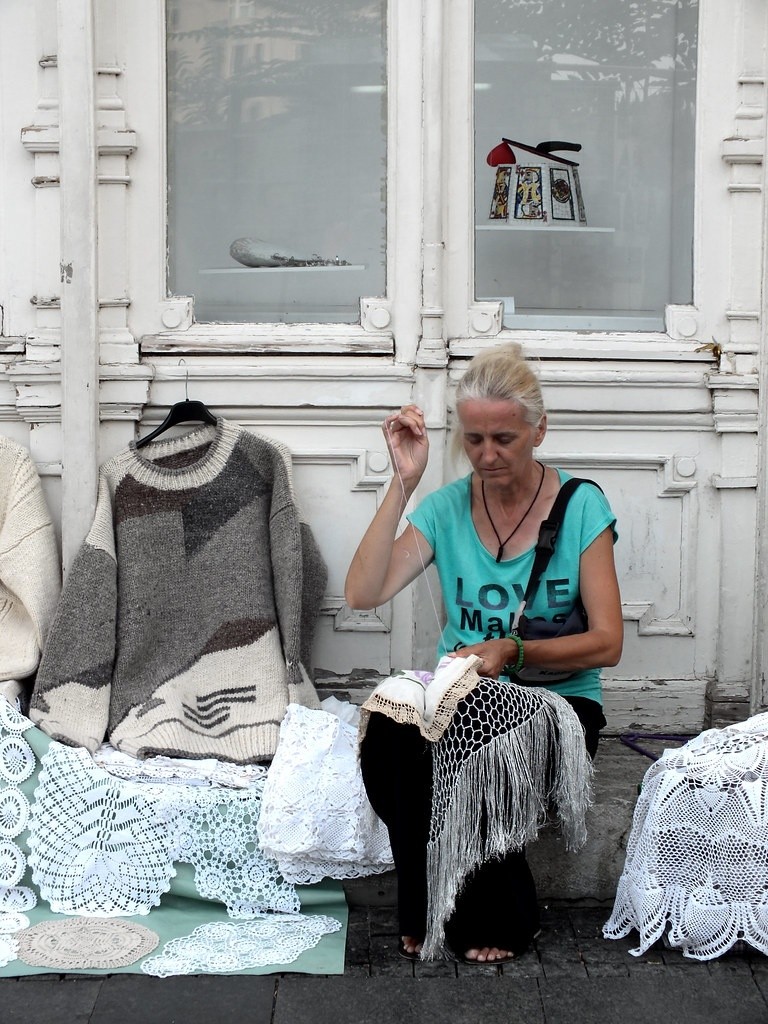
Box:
[136,359,217,449]
[621,733,691,761]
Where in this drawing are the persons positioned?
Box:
[346,346,623,962]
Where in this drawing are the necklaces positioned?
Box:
[481,460,544,564]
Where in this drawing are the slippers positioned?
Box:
[397,936,425,961]
[464,926,542,965]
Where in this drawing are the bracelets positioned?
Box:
[507,633,524,673]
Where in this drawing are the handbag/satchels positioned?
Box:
[507,477,604,687]
